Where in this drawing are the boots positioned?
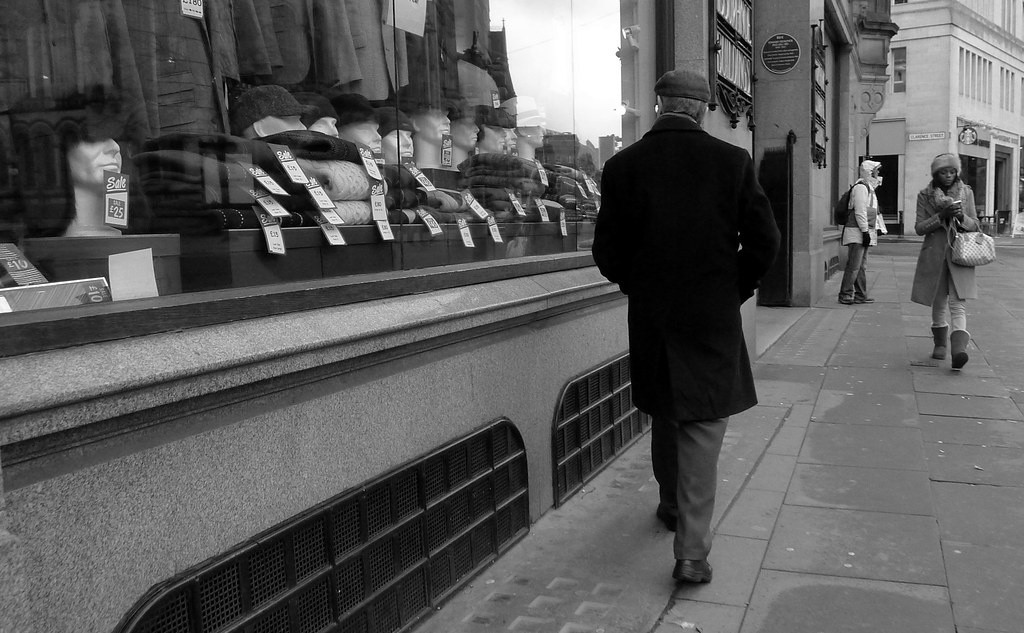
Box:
[950,330,970,368]
[931,322,949,359]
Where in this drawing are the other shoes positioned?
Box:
[838,297,853,304]
[853,297,874,303]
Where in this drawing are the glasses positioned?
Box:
[654,94,663,114]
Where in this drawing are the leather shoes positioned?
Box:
[673,558,712,583]
[657,502,677,531]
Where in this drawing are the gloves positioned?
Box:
[862,232,871,246]
[877,230,884,236]
[939,203,963,222]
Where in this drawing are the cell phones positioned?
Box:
[952,199,962,210]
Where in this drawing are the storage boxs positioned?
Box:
[190,221,577,289]
[17,233,182,298]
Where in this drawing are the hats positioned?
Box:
[229,82,547,133]
[872,164,882,177]
[931,153,962,178]
[654,70,710,102]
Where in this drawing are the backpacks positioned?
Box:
[835,180,870,225]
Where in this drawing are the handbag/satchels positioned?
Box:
[952,220,996,266]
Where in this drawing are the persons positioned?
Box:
[501,96,546,161]
[591,71,782,580]
[408,107,450,167]
[65,136,123,236]
[376,107,415,164]
[475,104,517,155]
[911,152,981,368]
[234,84,307,137]
[332,93,381,154]
[447,104,479,172]
[837,161,887,304]
[293,92,340,138]
